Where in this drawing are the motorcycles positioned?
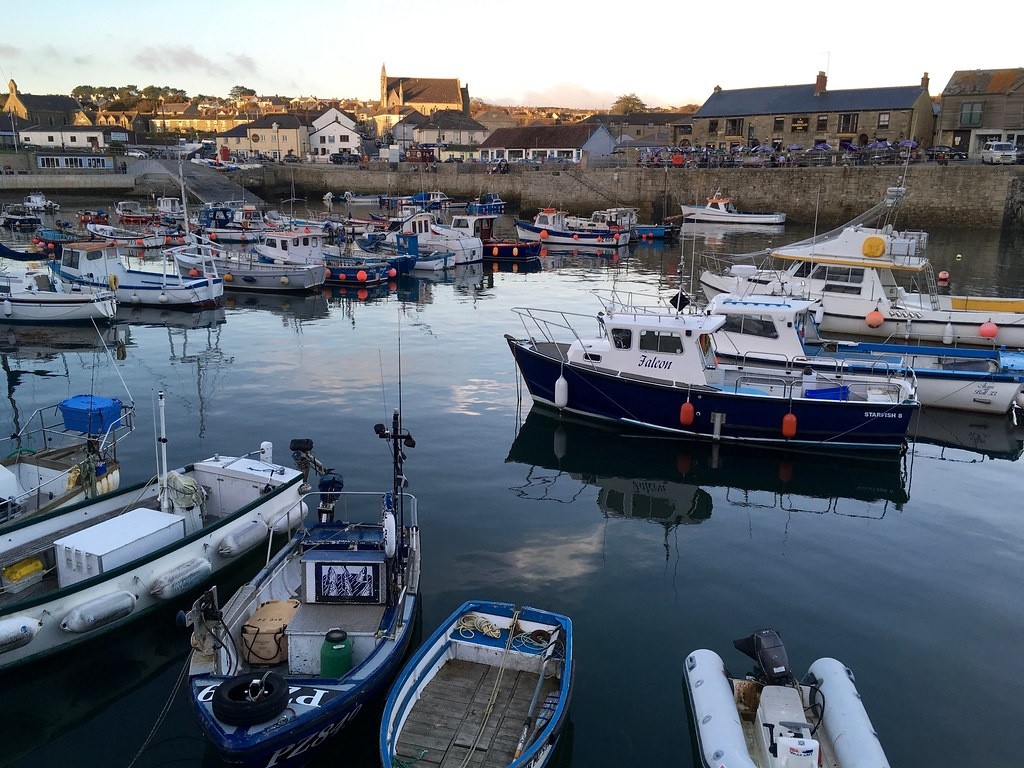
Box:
[936,150,949,166]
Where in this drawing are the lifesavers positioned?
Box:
[543,208,556,211]
[382,510,396,557]
[403,231,414,235]
[110,272,119,291]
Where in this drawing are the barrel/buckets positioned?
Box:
[319,630,352,679]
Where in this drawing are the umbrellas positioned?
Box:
[640,141,928,166]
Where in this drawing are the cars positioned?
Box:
[124,148,149,159]
[188,138,567,174]
[924,144,968,161]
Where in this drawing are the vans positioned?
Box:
[981,141,1017,164]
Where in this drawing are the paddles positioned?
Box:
[511,625,561,765]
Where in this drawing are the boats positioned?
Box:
[562,166,683,240]
[0,172,543,327]
[681,628,891,768]
[678,291,1023,419]
[379,600,573,768]
[680,187,786,224]
[513,206,630,248]
[694,109,1024,352]
[0,311,138,530]
[0,430,329,674]
[504,300,922,469]
[181,305,422,768]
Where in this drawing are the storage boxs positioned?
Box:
[240,597,301,666]
[804,385,851,402]
[58,394,124,434]
